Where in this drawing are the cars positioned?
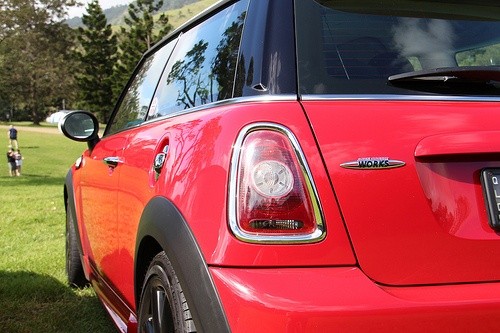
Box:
[61,1,498,331]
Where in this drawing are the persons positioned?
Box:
[8,125,19,149]
[6,148,24,177]
